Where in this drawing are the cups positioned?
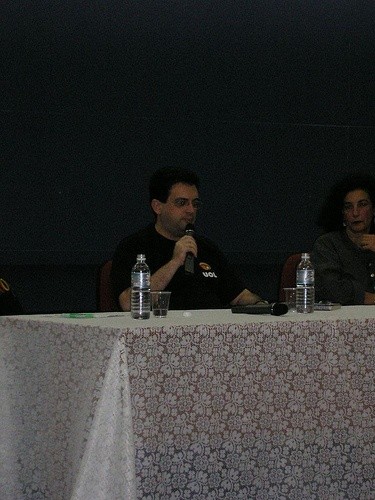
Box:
[150,291,171,317]
[284,288,297,311]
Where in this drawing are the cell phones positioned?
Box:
[313,303,341,311]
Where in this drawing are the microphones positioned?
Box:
[186,222,197,273]
[231,303,289,316]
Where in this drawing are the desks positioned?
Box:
[0,305,375,500]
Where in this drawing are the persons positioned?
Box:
[109,165,265,310]
[310,173,375,305]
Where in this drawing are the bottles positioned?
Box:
[296,253,315,314]
[131,254,150,320]
